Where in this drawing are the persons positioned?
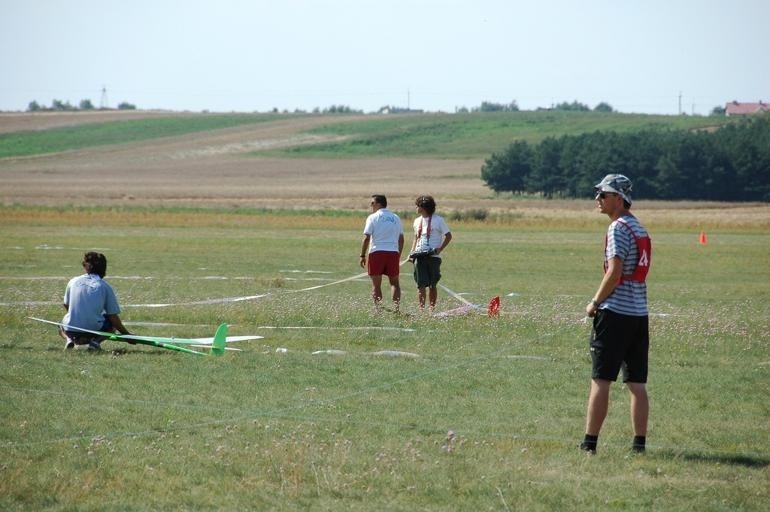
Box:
[359,194,405,318]
[56,249,137,353]
[405,193,452,315]
[573,172,652,461]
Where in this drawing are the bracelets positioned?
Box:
[591,298,600,307]
[360,254,366,258]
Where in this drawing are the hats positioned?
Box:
[594,173,633,205]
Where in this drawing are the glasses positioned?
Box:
[595,191,616,199]
[372,202,374,204]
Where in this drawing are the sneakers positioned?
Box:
[64,337,74,350]
[576,443,597,453]
[88,337,101,350]
